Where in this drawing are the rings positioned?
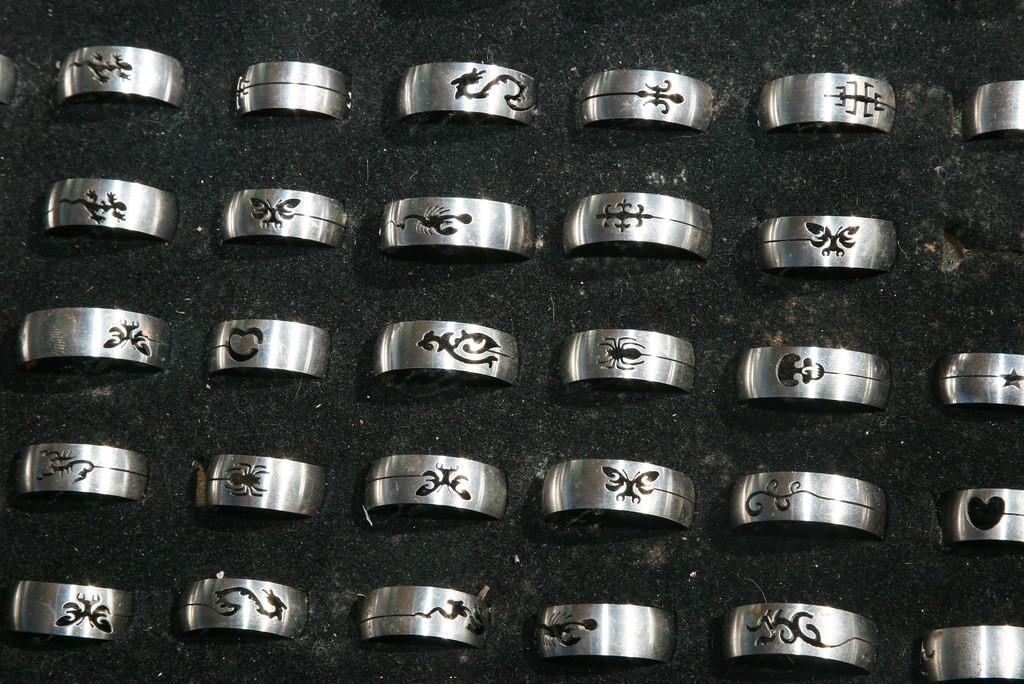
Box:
[364,455,507,517]
[920,80,1024,680]
[558,329,694,391]
[220,189,347,247]
[207,319,328,379]
[11,581,879,673]
[543,459,695,527]
[560,192,712,259]
[21,45,181,499]
[735,345,890,409]
[728,472,887,536]
[754,216,896,270]
[381,197,536,260]
[373,321,519,385]
[195,455,326,515]
[581,70,713,131]
[759,73,896,133]
[396,63,538,124]
[236,61,344,120]
